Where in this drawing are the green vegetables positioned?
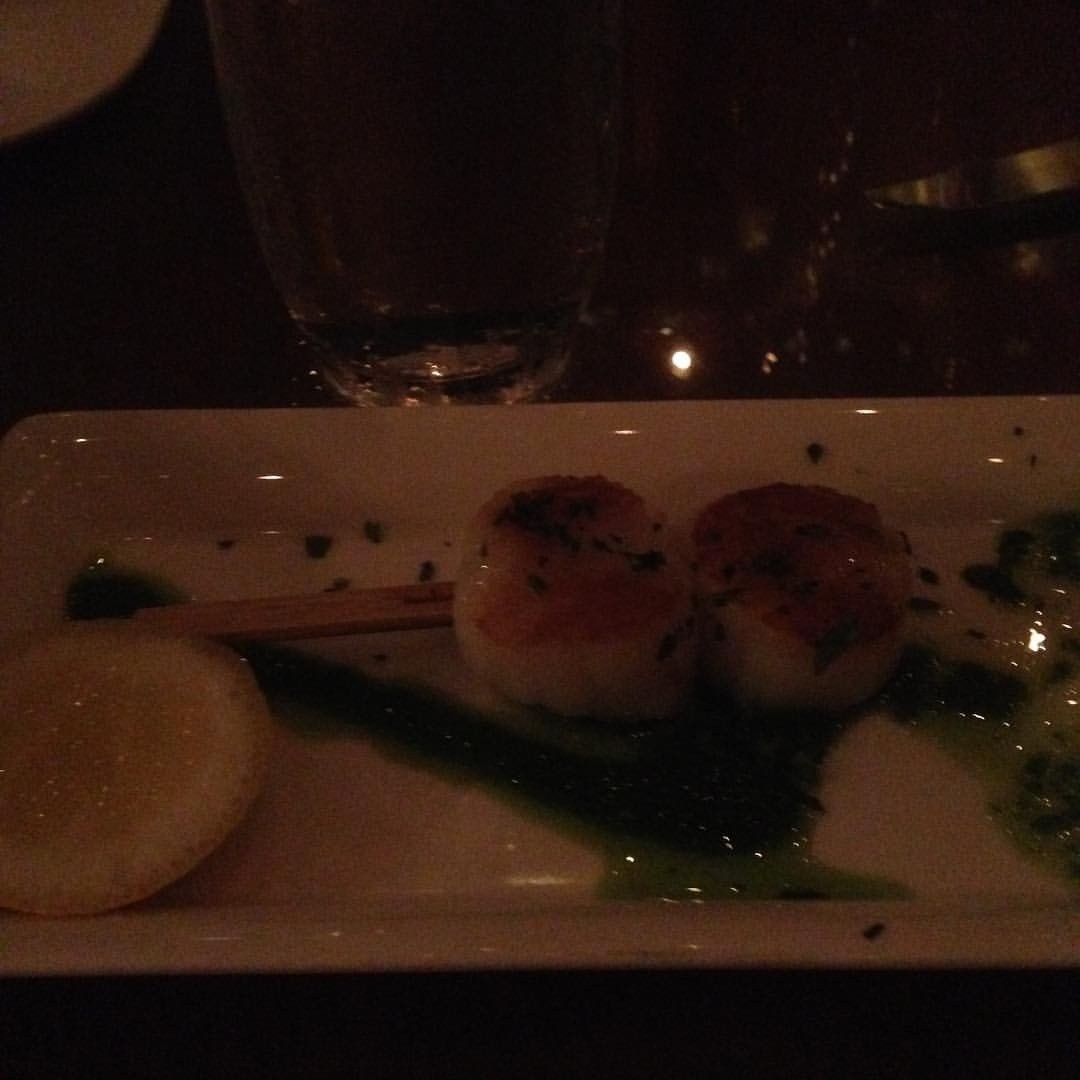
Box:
[62,490,1079,859]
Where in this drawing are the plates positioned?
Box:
[0,0,171,147]
[1,392,1080,975]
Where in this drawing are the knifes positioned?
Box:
[871,139,1080,210]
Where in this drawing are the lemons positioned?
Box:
[0,623,280,919]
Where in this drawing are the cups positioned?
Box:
[205,0,622,406]
